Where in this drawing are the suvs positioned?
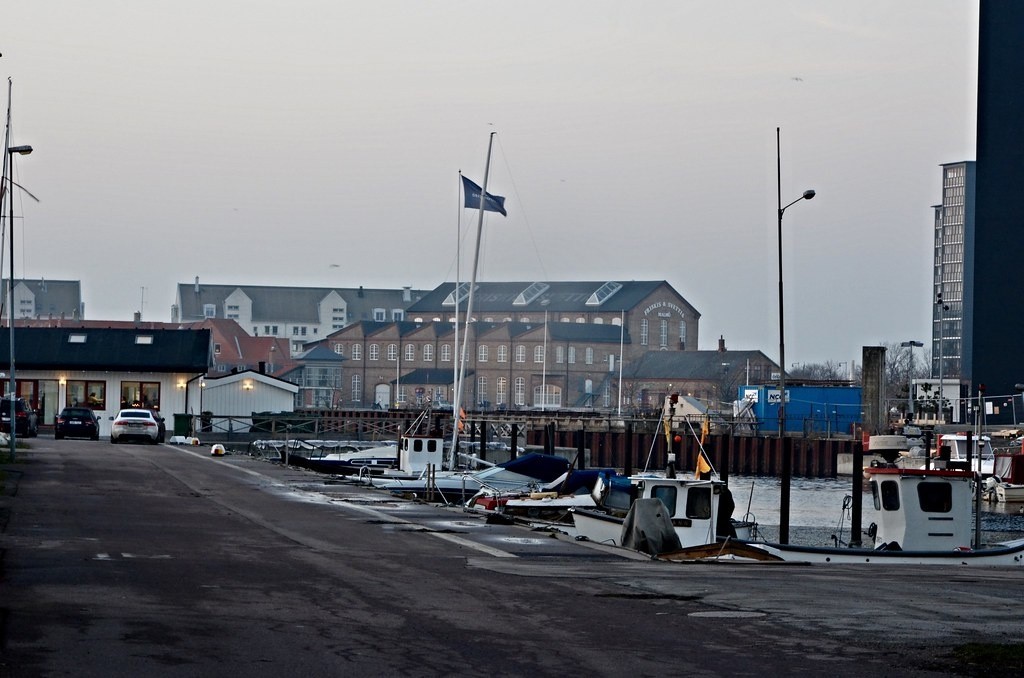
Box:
[0,396,38,438]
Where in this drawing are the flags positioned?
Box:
[461,174,507,217]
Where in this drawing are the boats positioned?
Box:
[898,428,1024,508]
[279,439,758,553]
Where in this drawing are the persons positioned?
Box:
[88,392,104,407]
[121,396,128,404]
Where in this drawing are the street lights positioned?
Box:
[899,338,923,422]
[4,142,34,457]
[777,190,816,540]
[936,292,949,424]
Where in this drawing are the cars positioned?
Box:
[54,407,101,441]
[110,408,166,444]
